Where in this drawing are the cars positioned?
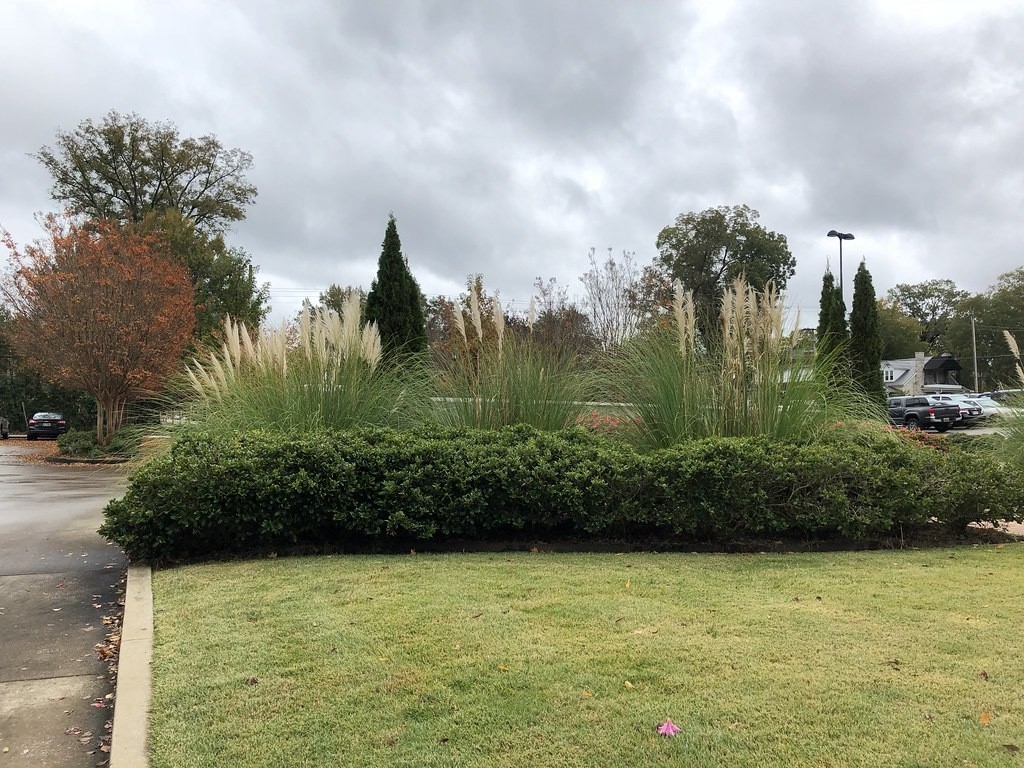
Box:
[0,416,11,441]
[931,395,985,428]
[959,396,1014,427]
[26,412,70,441]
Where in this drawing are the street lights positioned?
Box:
[826,229,856,302]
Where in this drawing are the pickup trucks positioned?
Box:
[885,395,963,433]
